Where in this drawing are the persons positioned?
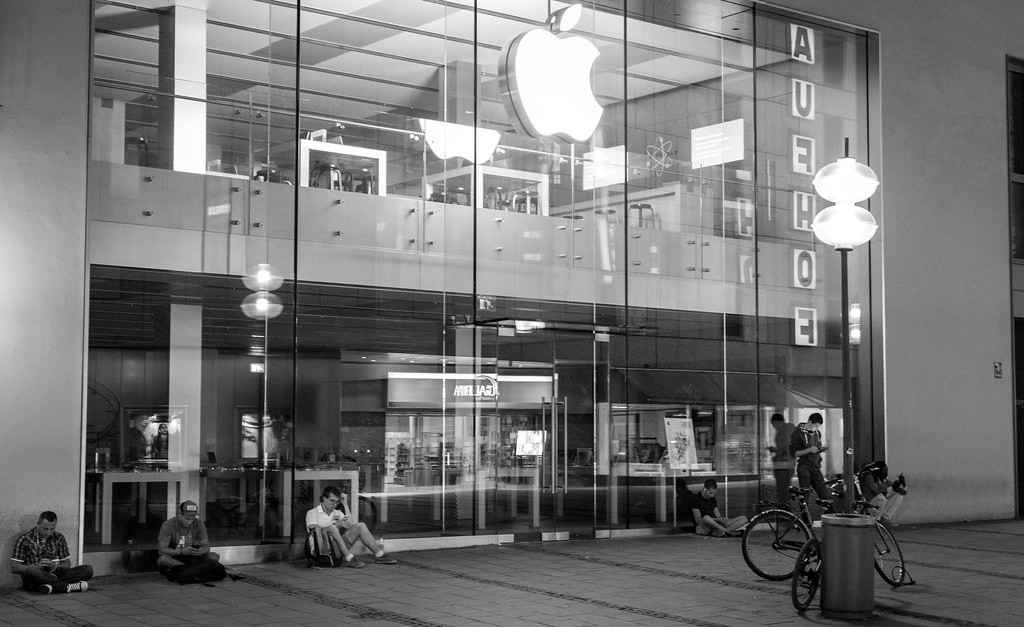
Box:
[157,500,221,568]
[10,511,93,595]
[690,478,748,537]
[126,413,150,463]
[766,413,798,489]
[789,413,836,531]
[305,486,397,568]
[152,424,177,460]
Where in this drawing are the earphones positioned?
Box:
[37,525,40,529]
[704,489,707,490]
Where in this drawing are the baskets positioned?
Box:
[758,483,788,504]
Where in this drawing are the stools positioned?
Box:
[564,215,582,220]
[124,135,149,167]
[628,203,655,227]
[431,183,541,213]
[253,160,377,195]
[597,210,615,221]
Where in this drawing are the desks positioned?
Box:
[364,461,541,531]
[389,164,550,216]
[250,139,387,198]
[93,97,126,165]
[551,184,713,236]
[607,462,667,525]
[260,469,359,538]
[96,471,190,546]
[199,468,248,513]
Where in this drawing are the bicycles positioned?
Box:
[740,457,908,610]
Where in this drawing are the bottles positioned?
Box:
[378,535,384,552]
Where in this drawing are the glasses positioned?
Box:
[326,497,340,505]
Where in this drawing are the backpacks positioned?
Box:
[304,526,343,568]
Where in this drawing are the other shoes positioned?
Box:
[712,530,725,537]
[345,556,366,568]
[375,553,398,564]
[730,530,744,536]
[880,518,900,527]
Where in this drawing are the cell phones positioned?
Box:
[343,515,349,519]
[191,545,201,549]
[52,559,60,566]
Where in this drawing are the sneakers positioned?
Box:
[38,584,52,594]
[66,581,88,593]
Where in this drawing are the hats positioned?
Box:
[178,500,199,516]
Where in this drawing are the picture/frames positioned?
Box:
[122,405,186,468]
[234,405,293,466]
